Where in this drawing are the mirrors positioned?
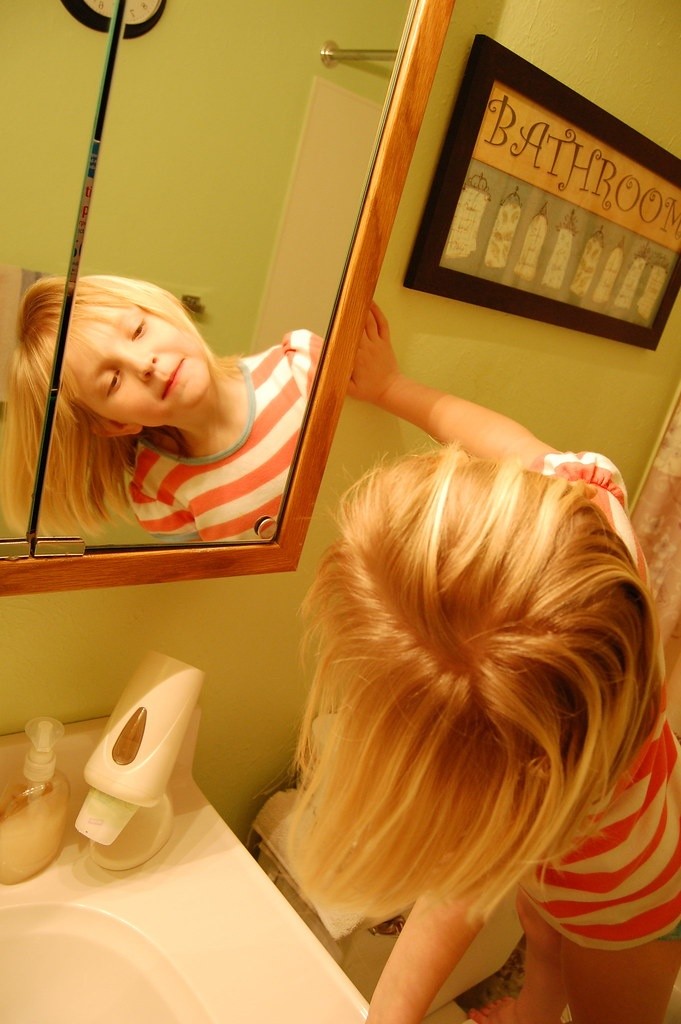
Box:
[0,0,456,598]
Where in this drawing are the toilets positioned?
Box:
[257,836,526,1024]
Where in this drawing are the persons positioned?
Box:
[290,301,681,1024]
[0,275,326,543]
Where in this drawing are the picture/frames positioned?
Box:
[400,32,681,352]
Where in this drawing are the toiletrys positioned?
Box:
[0,715,73,886]
[72,646,205,877]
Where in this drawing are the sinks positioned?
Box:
[0,898,220,1024]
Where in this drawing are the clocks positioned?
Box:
[60,0,166,40]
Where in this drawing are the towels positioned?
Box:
[251,788,366,940]
